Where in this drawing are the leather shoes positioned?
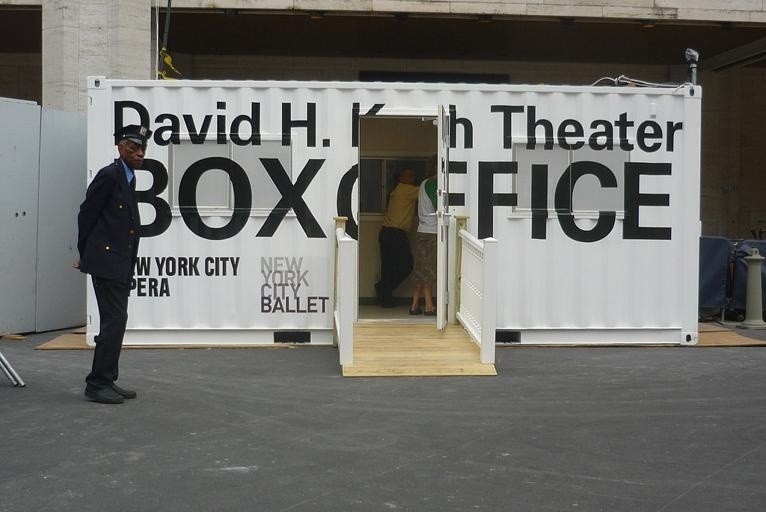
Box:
[85,383,136,403]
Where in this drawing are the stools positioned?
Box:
[0,335,26,387]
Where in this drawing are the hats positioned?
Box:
[116,125,152,146]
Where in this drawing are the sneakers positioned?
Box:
[409,307,437,315]
[375,283,395,307]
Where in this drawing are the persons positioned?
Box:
[71,124,153,403]
[374,164,421,307]
[407,152,437,316]
